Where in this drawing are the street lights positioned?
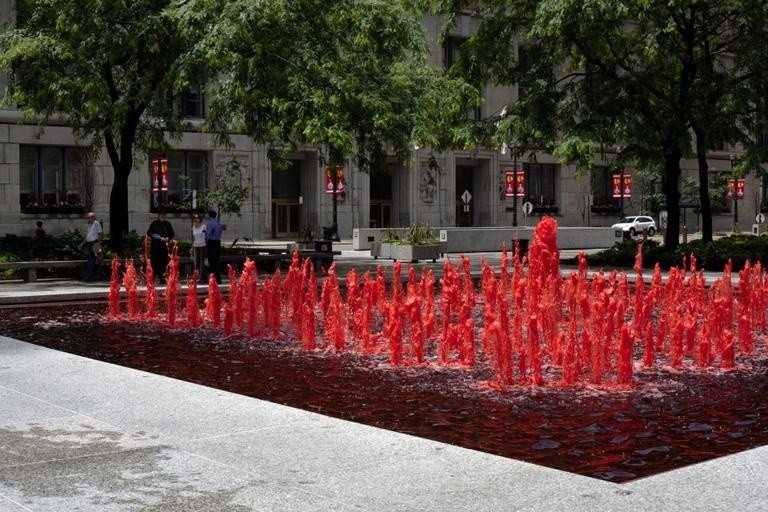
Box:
[613,141,627,216]
[505,138,519,227]
[727,150,739,234]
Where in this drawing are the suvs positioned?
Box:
[610,216,657,237]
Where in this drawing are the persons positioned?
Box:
[35,221,46,235]
[205,210,222,284]
[191,213,207,283]
[78,212,102,281]
[147,207,176,283]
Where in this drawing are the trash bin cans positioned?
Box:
[314,241,332,273]
[511,239,529,268]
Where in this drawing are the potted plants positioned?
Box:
[368,217,449,263]
[286,223,327,255]
